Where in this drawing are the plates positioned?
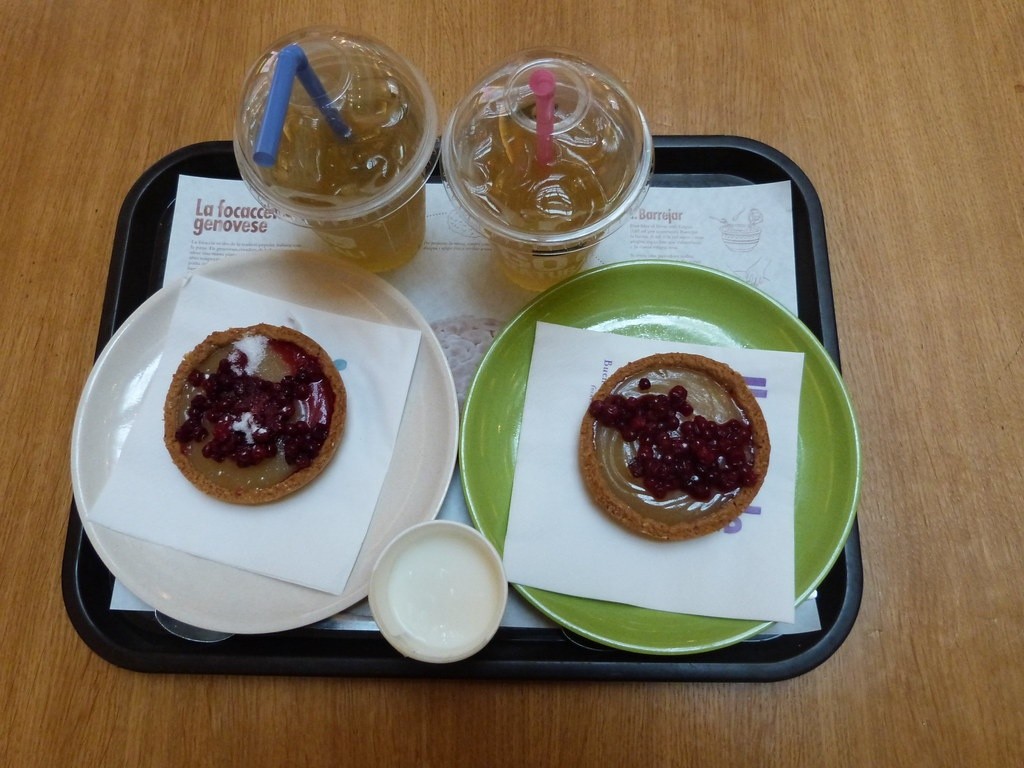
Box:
[72,251,460,634]
[460,261,859,655]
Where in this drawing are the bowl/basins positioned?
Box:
[368,521,507,663]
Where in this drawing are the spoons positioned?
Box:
[155,611,379,643]
[562,589,817,651]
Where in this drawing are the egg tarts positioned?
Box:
[579,351,770,540]
[163,323,347,503]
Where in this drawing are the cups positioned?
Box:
[232,25,438,272]
[442,47,653,291]
